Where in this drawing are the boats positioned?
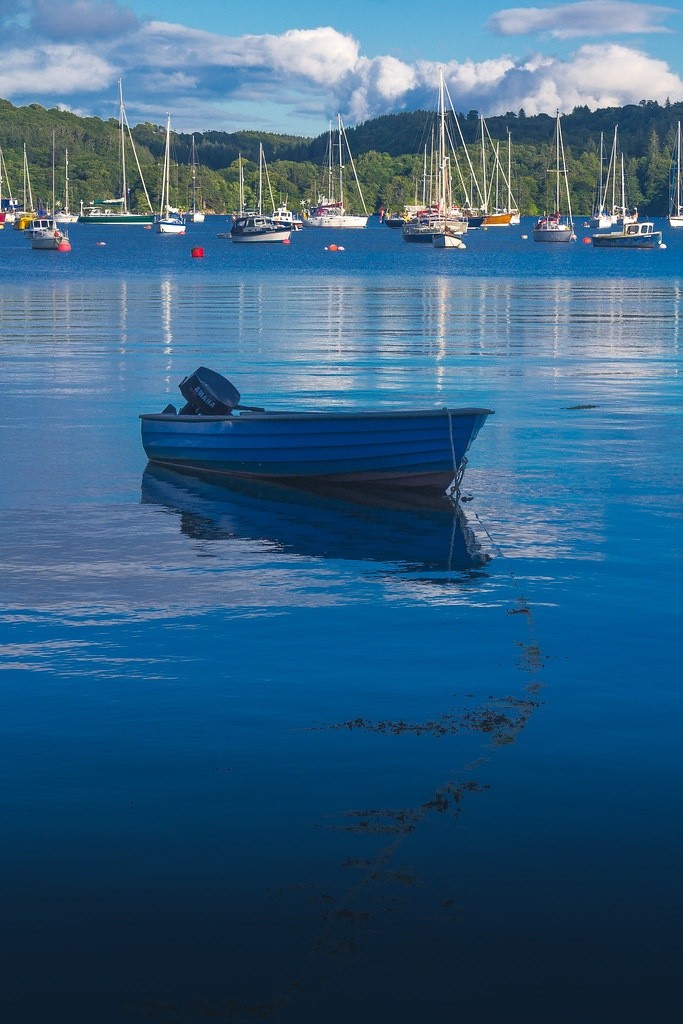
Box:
[271,192,304,230]
[433,220,462,248]
[136,366,496,493]
[24,218,59,238]
[138,461,493,571]
[230,216,292,243]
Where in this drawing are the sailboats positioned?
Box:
[385,66,520,242]
[0,143,79,230]
[232,141,275,224]
[301,112,370,229]
[589,131,612,229]
[157,112,187,233]
[610,124,639,226]
[532,108,575,242]
[78,77,157,225]
[32,129,64,250]
[189,135,206,223]
[593,152,663,247]
[668,121,683,227]
